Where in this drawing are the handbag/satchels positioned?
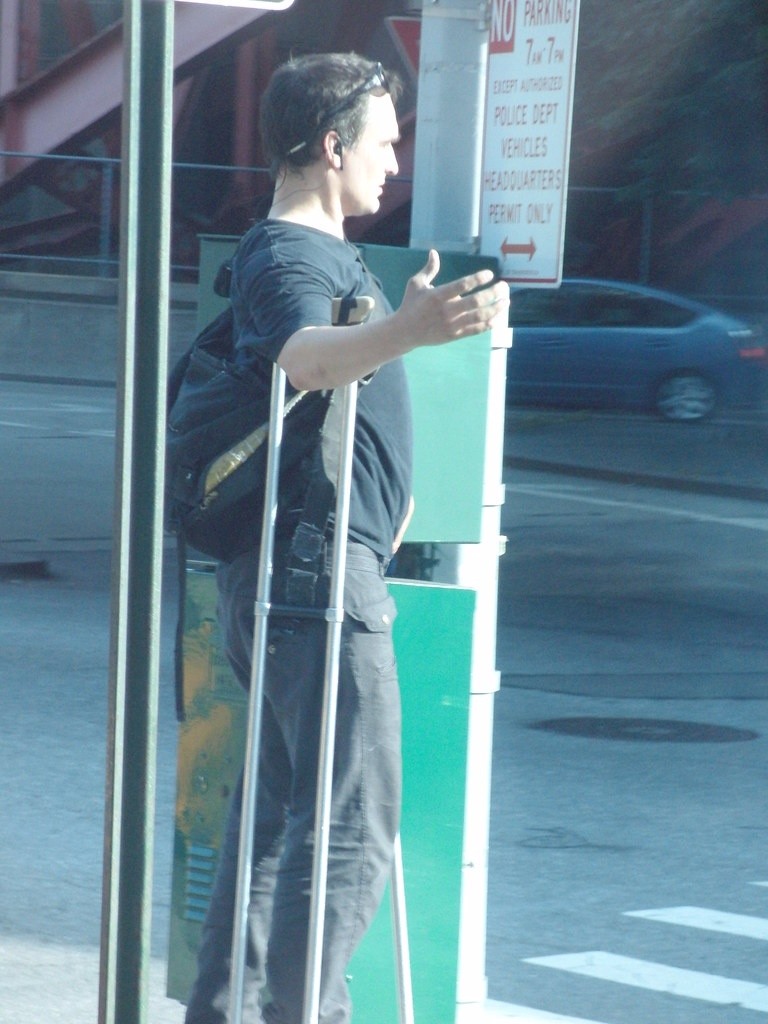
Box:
[165,234,387,563]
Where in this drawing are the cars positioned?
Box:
[503,274,768,420]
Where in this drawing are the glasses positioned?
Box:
[314,61,391,137]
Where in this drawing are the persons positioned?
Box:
[185,52,510,1024]
